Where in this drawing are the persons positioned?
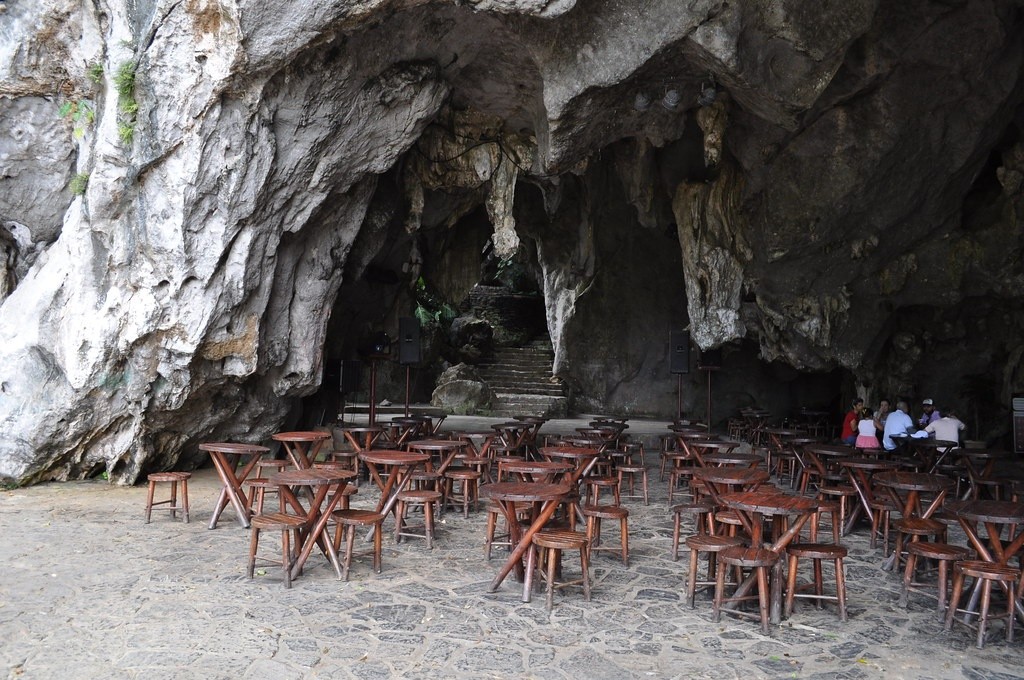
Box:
[840,398,966,453]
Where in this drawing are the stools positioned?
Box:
[246,510,307,586]
[258,457,287,476]
[946,559,1019,650]
[145,470,192,526]
[440,469,485,517]
[465,455,489,488]
[682,532,747,615]
[531,529,594,613]
[900,540,976,623]
[709,544,781,634]
[243,478,289,521]
[481,406,1024,577]
[336,509,385,581]
[406,470,447,516]
[391,489,443,552]
[332,447,361,489]
[782,540,851,620]
[315,481,360,512]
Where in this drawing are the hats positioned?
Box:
[921,399,935,406]
[910,430,929,439]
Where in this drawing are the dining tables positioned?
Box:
[394,413,450,444]
[486,478,584,603]
[197,441,270,531]
[716,490,822,621]
[943,496,1024,628]
[456,429,498,484]
[343,426,390,479]
[272,430,333,495]
[378,420,410,453]
[406,437,468,511]
[266,464,359,585]
